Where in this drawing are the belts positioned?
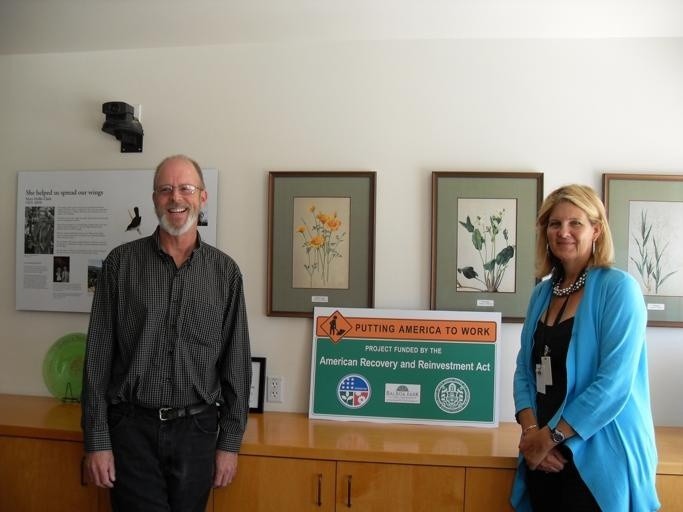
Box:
[124,399,213,422]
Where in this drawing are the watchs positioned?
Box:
[549,422,565,443]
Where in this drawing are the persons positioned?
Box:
[508,186,662,511]
[81,155,252,512]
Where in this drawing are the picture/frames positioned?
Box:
[266,170,377,319]
[431,170,543,325]
[598,172,682,328]
[246,357,266,414]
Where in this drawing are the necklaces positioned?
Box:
[547,269,591,296]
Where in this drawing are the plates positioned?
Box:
[40,332,88,402]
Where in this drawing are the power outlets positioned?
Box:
[266,373,282,404]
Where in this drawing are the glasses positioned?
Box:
[151,182,207,197]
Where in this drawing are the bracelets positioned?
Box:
[522,424,538,432]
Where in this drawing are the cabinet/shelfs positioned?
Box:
[214,445,465,512]
[465,469,683,512]
[0,435,213,512]
